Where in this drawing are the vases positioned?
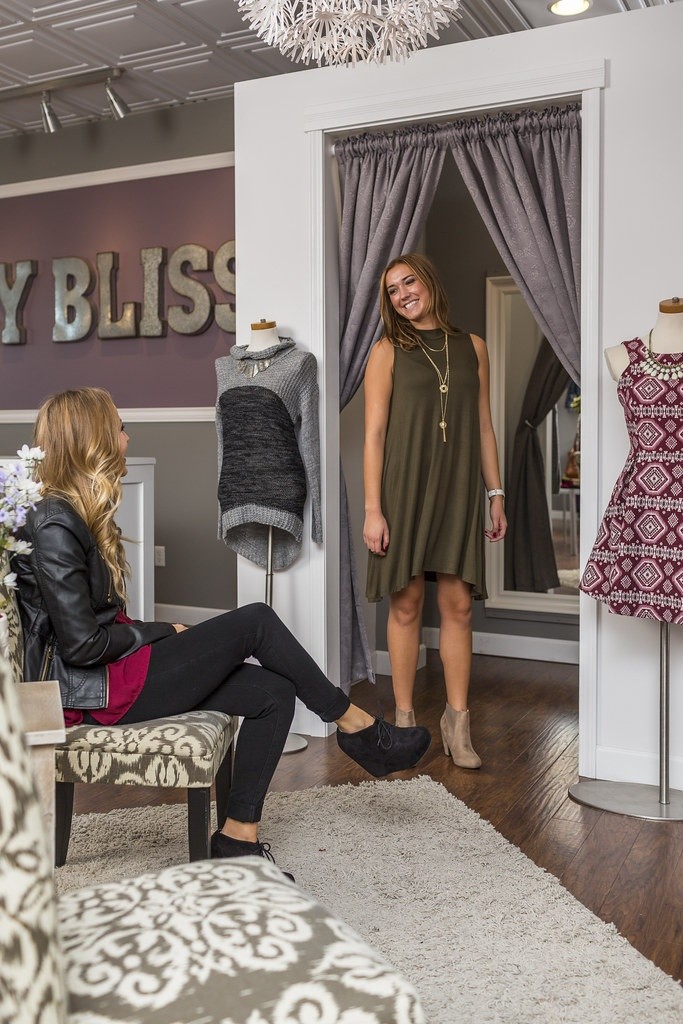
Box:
[0,613,11,659]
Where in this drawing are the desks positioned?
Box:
[15,678,67,871]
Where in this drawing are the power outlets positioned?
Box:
[155,546,165,567]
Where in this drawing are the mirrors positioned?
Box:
[484,275,580,617]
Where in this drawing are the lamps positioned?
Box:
[104,78,131,118]
[39,90,62,133]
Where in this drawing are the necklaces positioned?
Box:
[238,347,296,378]
[415,330,450,443]
[640,328,683,381]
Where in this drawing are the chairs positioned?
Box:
[2,543,240,871]
[1,654,422,1024]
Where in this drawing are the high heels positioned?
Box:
[210,829,294,882]
[440,701,482,768]
[336,703,431,778]
[394,706,416,728]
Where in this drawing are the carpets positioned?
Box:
[55,775,683,1023]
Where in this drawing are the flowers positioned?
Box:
[0,445,45,618]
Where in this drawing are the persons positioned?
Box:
[214,319,324,570]
[578,297,683,625]
[364,253,509,767]
[8,385,432,882]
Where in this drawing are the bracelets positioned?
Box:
[487,488,505,500]
